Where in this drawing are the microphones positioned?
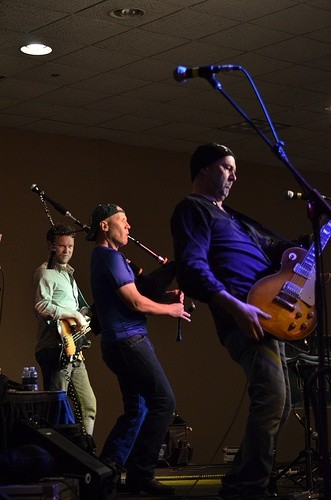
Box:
[295,413,319,440]
[30,183,71,217]
[172,63,242,83]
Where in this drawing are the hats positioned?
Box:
[188,142,234,182]
[85,203,126,242]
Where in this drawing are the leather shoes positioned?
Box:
[123,473,176,497]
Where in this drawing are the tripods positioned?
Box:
[201,64,330,500]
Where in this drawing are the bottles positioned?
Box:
[22,367,32,391]
[28,367,38,390]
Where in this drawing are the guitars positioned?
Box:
[55,310,91,360]
[245,218,330,341]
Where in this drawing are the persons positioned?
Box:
[170,142,330,500]
[85,203,192,499]
[32,225,98,438]
